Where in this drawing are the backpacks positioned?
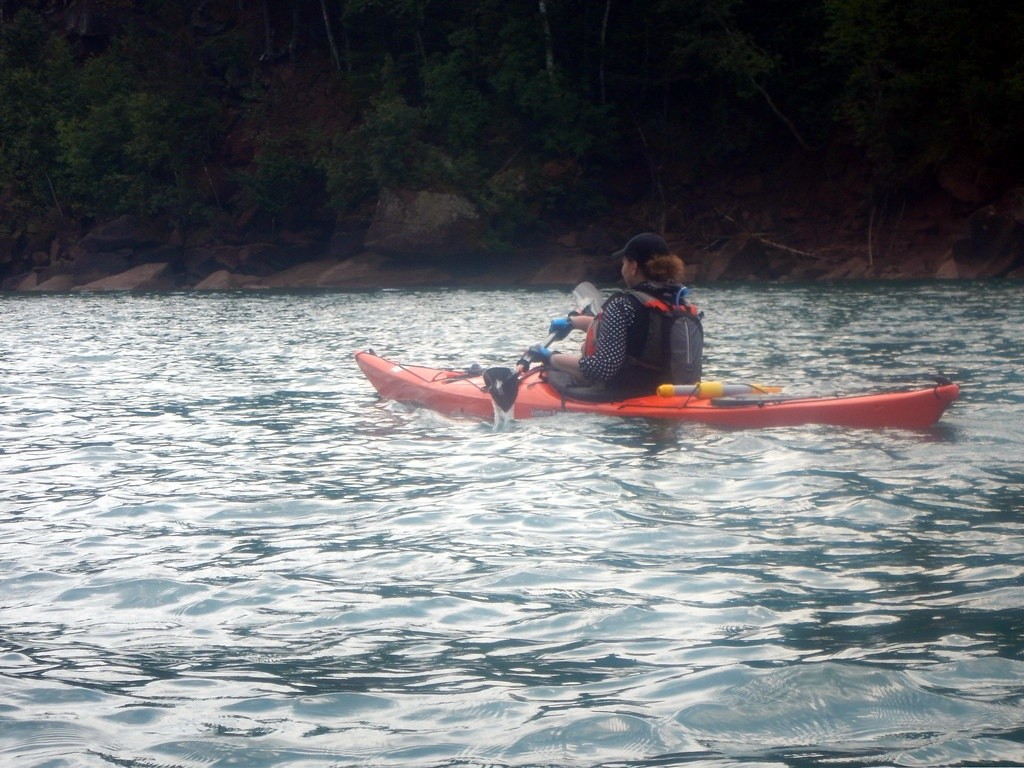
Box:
[638,303,704,392]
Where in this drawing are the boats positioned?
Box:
[351,347,959,435]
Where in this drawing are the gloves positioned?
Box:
[548,317,573,342]
[528,343,559,367]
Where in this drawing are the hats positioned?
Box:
[611,232,671,268]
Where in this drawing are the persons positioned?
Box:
[525,232,703,400]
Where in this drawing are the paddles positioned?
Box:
[482,281,604,413]
[657,381,808,398]
